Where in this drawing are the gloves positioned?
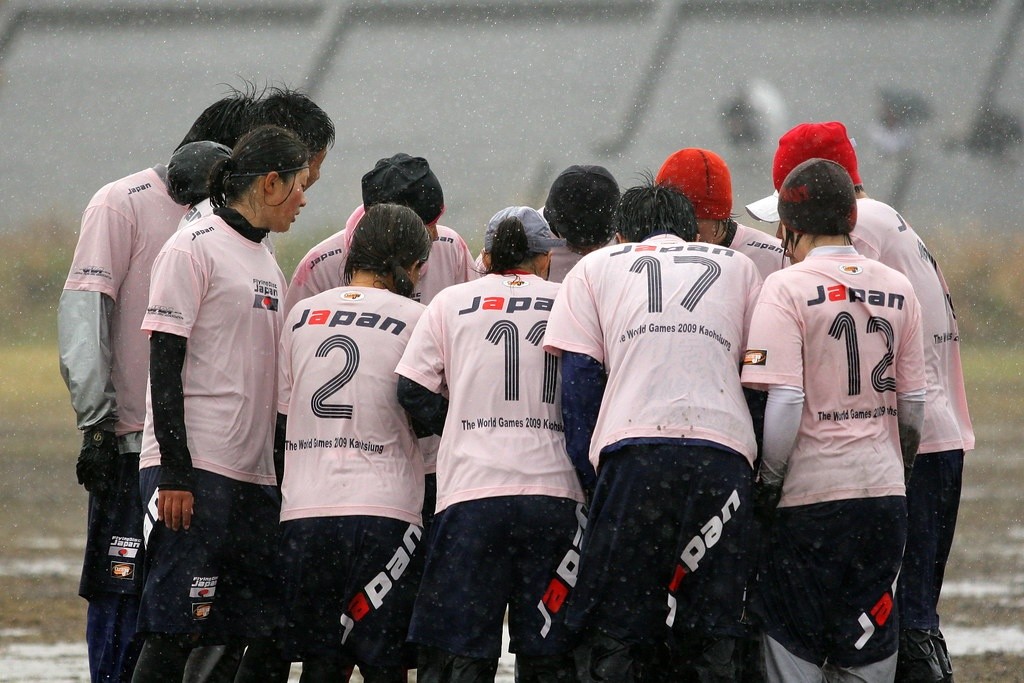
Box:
[76,427,122,499]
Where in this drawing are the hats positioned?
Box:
[744,139,858,219]
[544,164,620,244]
[653,148,733,220]
[773,121,860,190]
[777,160,857,233]
[486,206,567,255]
[361,154,444,225]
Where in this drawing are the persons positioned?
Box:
[739,120,975,683]
[230,152,479,683]
[58,89,335,683]
[396,148,792,683]
